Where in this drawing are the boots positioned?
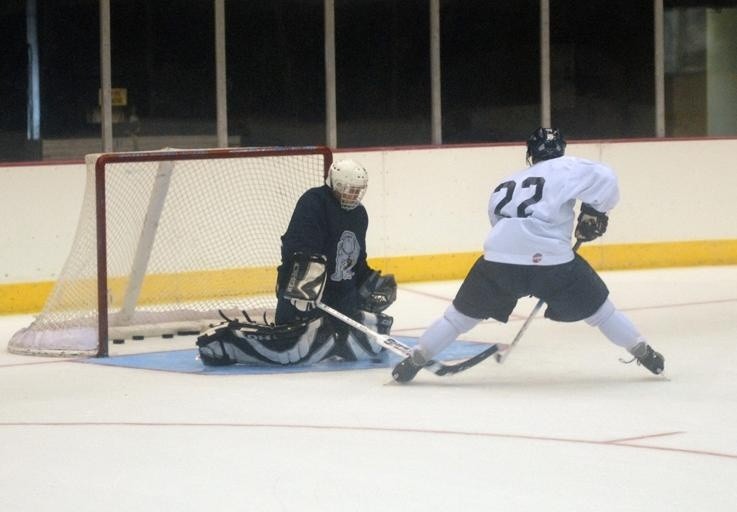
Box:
[632,341,664,376]
[394,350,428,381]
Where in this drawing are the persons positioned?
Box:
[197,159,387,368]
[391,128,665,384]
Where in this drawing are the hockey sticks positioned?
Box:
[491,241,582,368]
[316,303,505,376]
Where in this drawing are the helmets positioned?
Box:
[524,127,566,164]
[324,159,368,210]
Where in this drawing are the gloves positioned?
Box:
[575,200,609,241]
